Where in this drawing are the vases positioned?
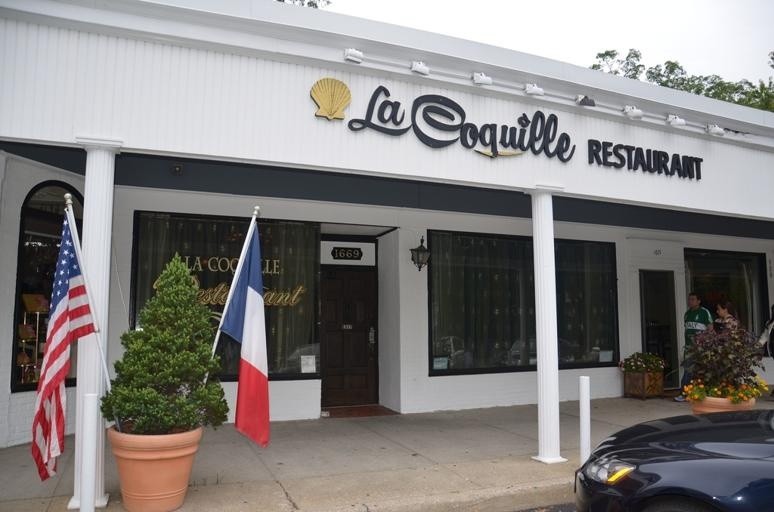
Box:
[691,395,756,416]
[622,371,664,401]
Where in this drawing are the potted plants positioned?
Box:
[100,252,229,512]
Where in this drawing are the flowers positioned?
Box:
[682,323,768,404]
[620,353,666,371]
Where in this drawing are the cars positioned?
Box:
[267,342,320,375]
[568,405,773,512]
[433,331,474,370]
[498,333,571,366]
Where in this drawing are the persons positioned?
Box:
[671,290,715,403]
[751,303,774,402]
[709,302,744,391]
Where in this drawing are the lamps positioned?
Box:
[341,44,728,140]
[408,235,432,272]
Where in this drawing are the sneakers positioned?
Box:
[673,394,687,403]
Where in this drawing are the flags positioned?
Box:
[215,206,272,446]
[28,191,97,483]
